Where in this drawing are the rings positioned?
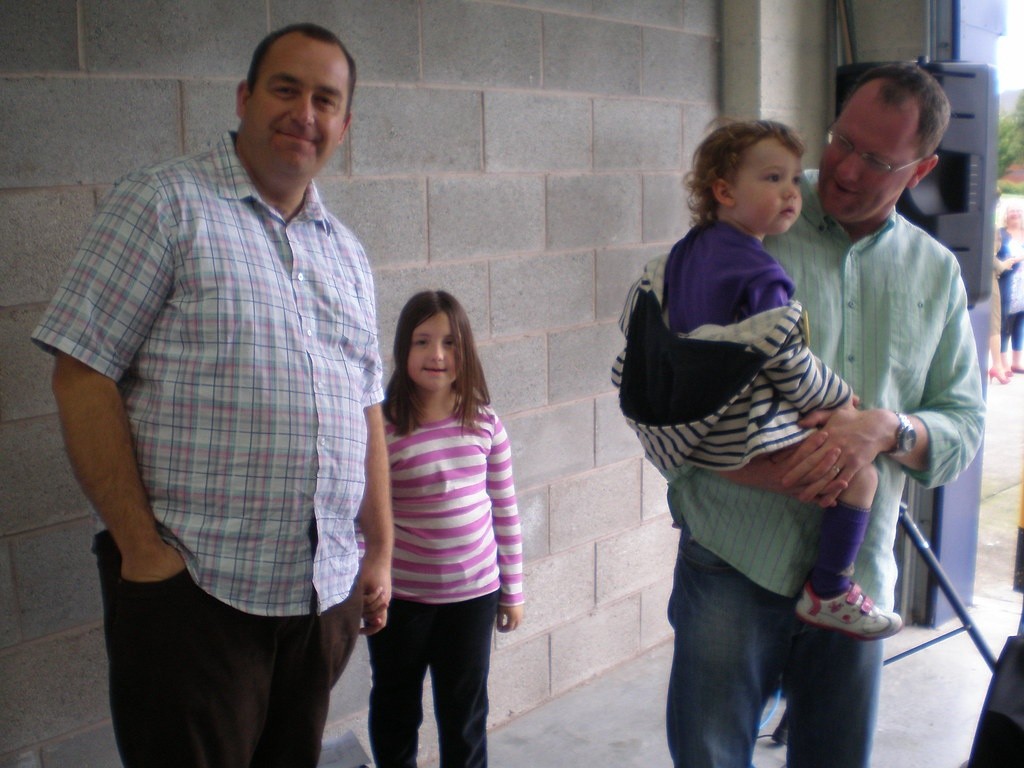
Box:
[834,464,840,473]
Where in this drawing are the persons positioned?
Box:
[995,198,1024,378]
[34,20,395,768]
[989,186,1010,383]
[663,61,987,767]
[611,115,904,644]
[361,291,522,768]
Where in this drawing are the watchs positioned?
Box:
[887,410,917,458]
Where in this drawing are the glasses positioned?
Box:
[824,119,927,175]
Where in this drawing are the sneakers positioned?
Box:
[796,582,903,641]
